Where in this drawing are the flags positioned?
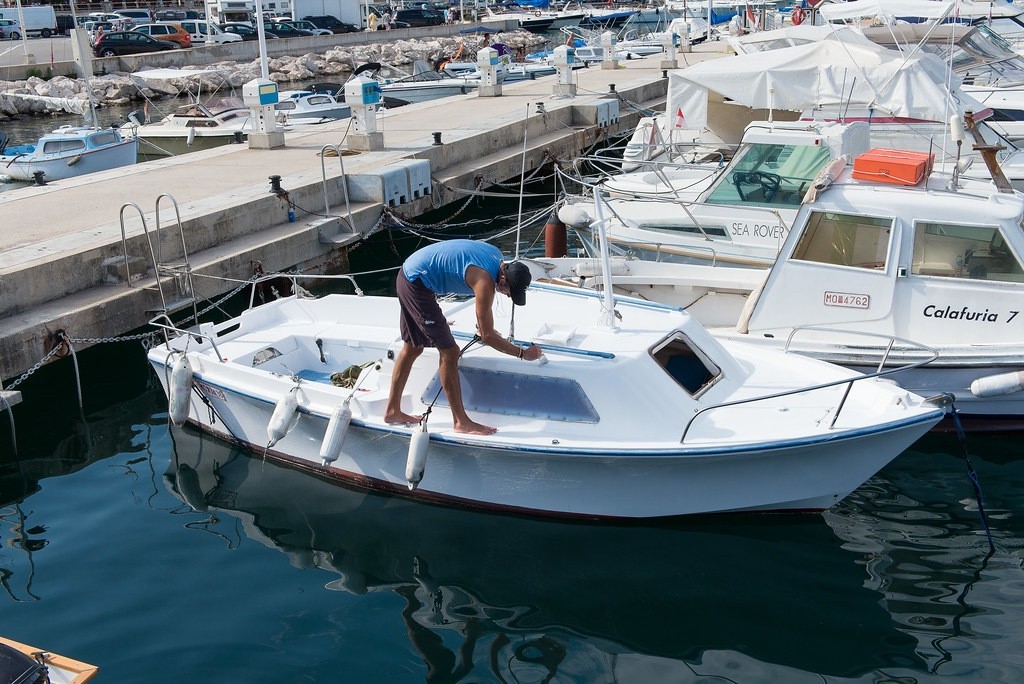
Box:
[747,4,755,24]
[675,107,684,129]
[644,122,661,160]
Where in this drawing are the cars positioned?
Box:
[128,22,193,48]
[0,20,22,39]
[90,31,181,58]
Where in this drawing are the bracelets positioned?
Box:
[517,348,524,360]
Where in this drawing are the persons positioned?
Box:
[368,9,378,31]
[384,239,542,436]
[516,47,525,63]
[482,33,489,47]
[97,26,103,38]
[112,20,124,32]
[382,9,393,30]
[444,5,454,24]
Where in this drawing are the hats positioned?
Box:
[503,262,532,306]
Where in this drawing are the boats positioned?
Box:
[146,272,946,522]
[0,0,1024,285]
[504,255,1024,435]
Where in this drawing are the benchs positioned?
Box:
[195,321,400,368]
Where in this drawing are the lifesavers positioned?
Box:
[535,11,541,17]
[791,9,806,25]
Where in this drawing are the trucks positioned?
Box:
[0,6,59,37]
